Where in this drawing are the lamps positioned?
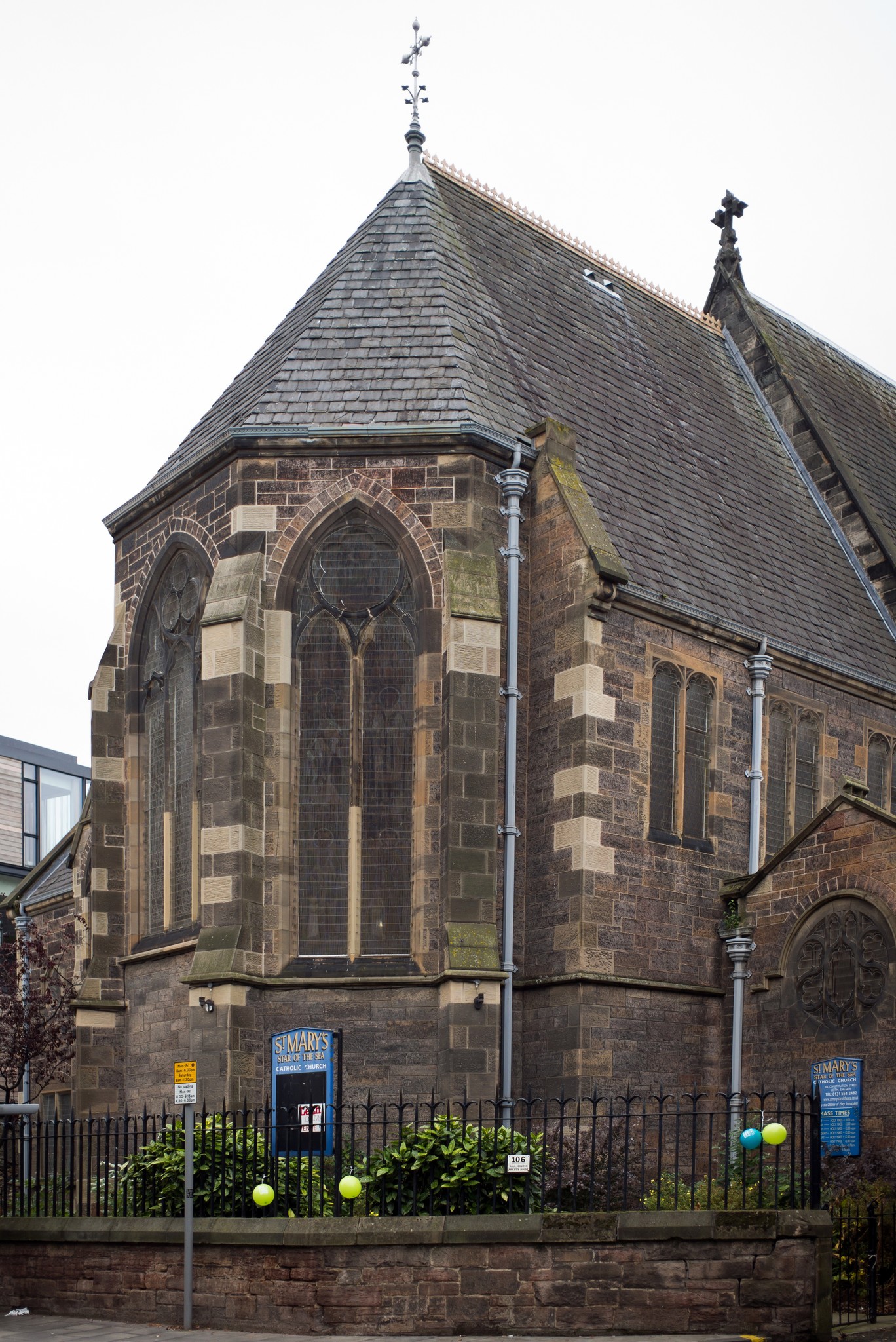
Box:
[474,994,484,1010]
[199,997,213,1012]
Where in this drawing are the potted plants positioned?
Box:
[717,900,754,938]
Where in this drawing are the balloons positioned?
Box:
[739,1128,762,1149]
[339,1176,361,1199]
[761,1123,787,1145]
[252,1184,275,1206]
